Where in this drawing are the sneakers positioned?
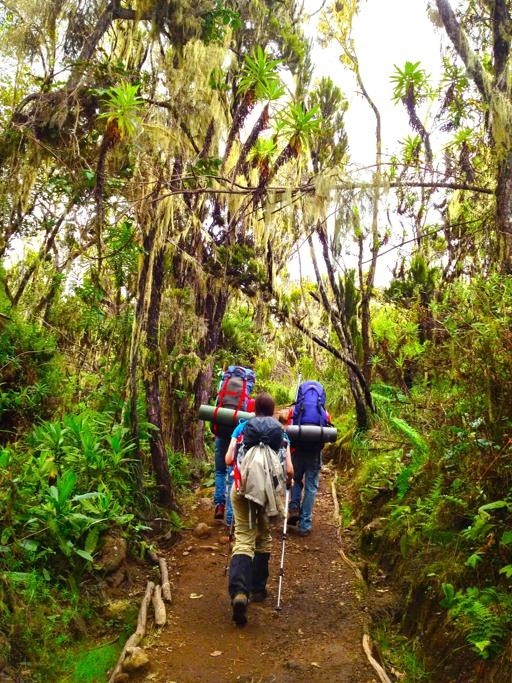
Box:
[287,508,311,537]
[231,589,267,629]
[214,503,225,520]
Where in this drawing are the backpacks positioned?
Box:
[209,365,256,441]
[235,415,287,480]
[290,380,327,449]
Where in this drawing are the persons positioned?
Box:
[287,381,330,537]
[210,366,256,535]
[226,392,295,626]
